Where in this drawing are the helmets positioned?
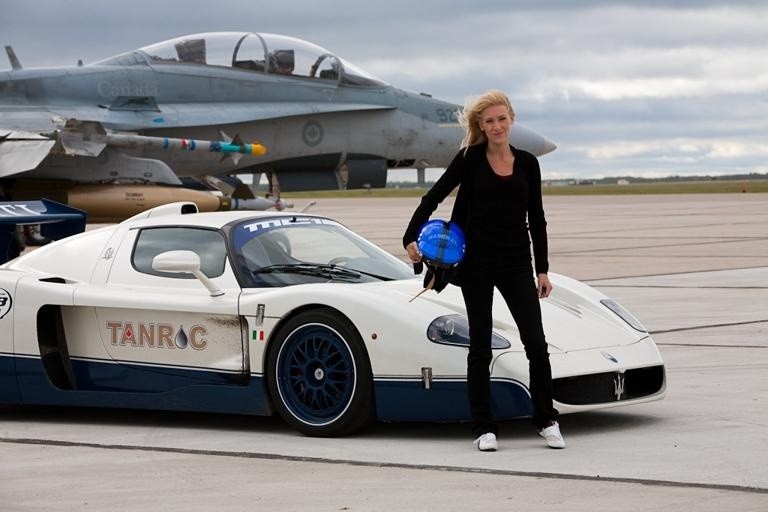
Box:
[415,218,469,269]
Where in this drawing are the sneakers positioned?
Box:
[475,432,499,453]
[534,419,566,449]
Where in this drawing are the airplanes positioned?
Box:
[0,30,556,224]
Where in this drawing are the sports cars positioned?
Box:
[0,211,667,438]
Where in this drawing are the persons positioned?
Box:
[402,90,566,451]
